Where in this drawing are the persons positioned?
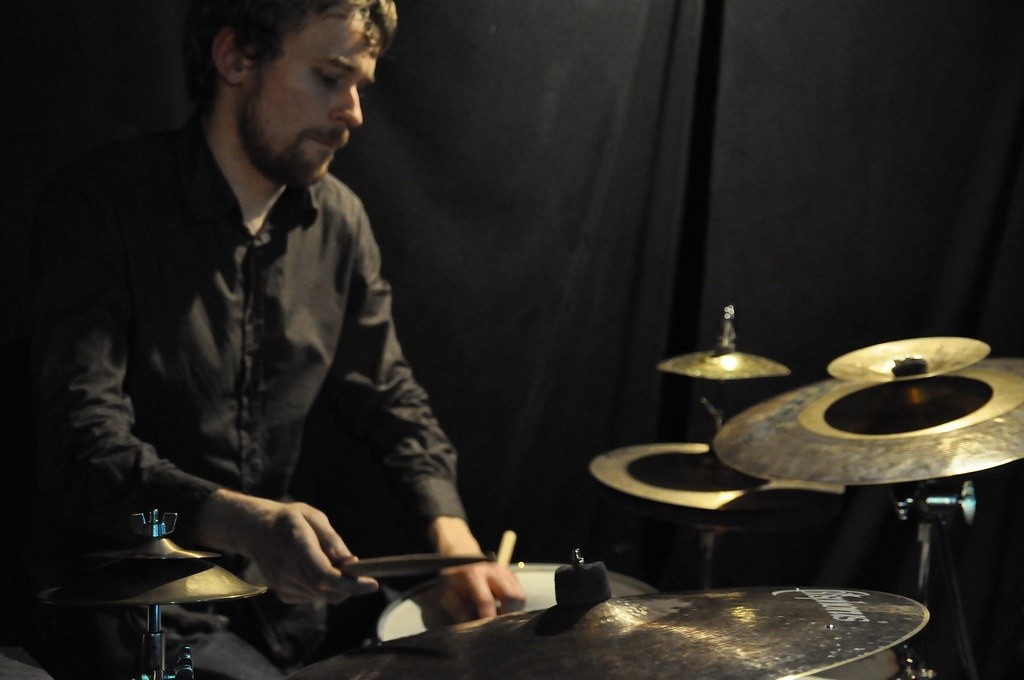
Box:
[16,0,526,680]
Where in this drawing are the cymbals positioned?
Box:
[823,334,993,386]
[34,553,271,613]
[709,354,1024,493]
[655,350,795,384]
[72,539,224,566]
[281,584,933,680]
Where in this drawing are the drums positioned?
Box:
[363,558,663,645]
[788,643,929,680]
[577,439,773,590]
